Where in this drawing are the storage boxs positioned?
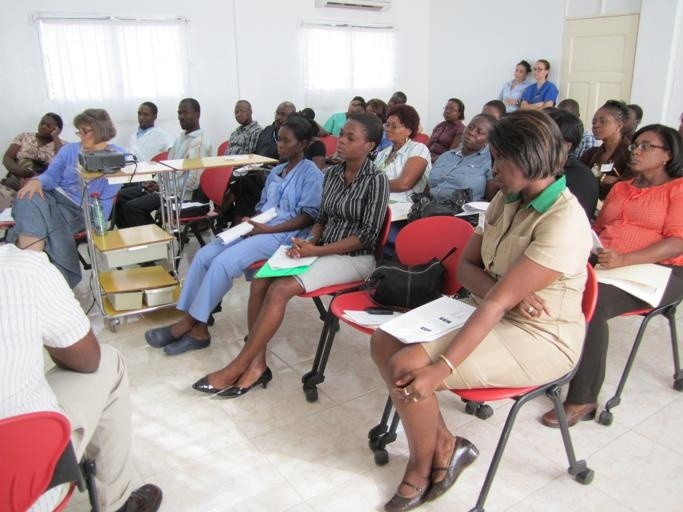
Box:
[99,266,178,311]
[92,223,174,269]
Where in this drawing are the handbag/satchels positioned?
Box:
[407,188,473,225]
[369,256,448,312]
[157,199,211,218]
[4,158,50,192]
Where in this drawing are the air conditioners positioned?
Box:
[314,0,392,13]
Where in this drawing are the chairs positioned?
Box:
[169,165,234,277]
[599,296,682,427]
[304,215,475,451]
[216,140,229,156]
[73,229,92,270]
[314,136,340,158]
[374,261,599,512]
[242,259,266,281]
[298,205,392,383]
[0,410,100,512]
[410,132,430,146]
[150,151,170,162]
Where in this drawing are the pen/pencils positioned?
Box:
[613,167,621,177]
[306,234,316,241]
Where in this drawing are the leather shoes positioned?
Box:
[192,362,272,397]
[384,435,480,512]
[91,483,163,512]
[542,397,599,428]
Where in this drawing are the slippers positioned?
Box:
[145,321,210,355]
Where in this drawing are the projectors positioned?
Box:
[77,149,126,175]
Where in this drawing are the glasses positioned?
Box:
[628,143,668,152]
[383,123,404,130]
[75,128,93,138]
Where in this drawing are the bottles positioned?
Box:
[88,192,109,238]
